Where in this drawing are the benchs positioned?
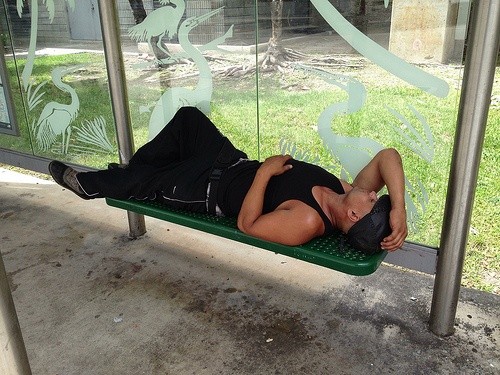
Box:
[106,194,388,276]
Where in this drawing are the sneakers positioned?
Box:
[48,160,93,201]
[108,160,132,174]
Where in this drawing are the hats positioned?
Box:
[345,192,392,257]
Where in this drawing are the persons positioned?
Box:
[47,106,409,252]
